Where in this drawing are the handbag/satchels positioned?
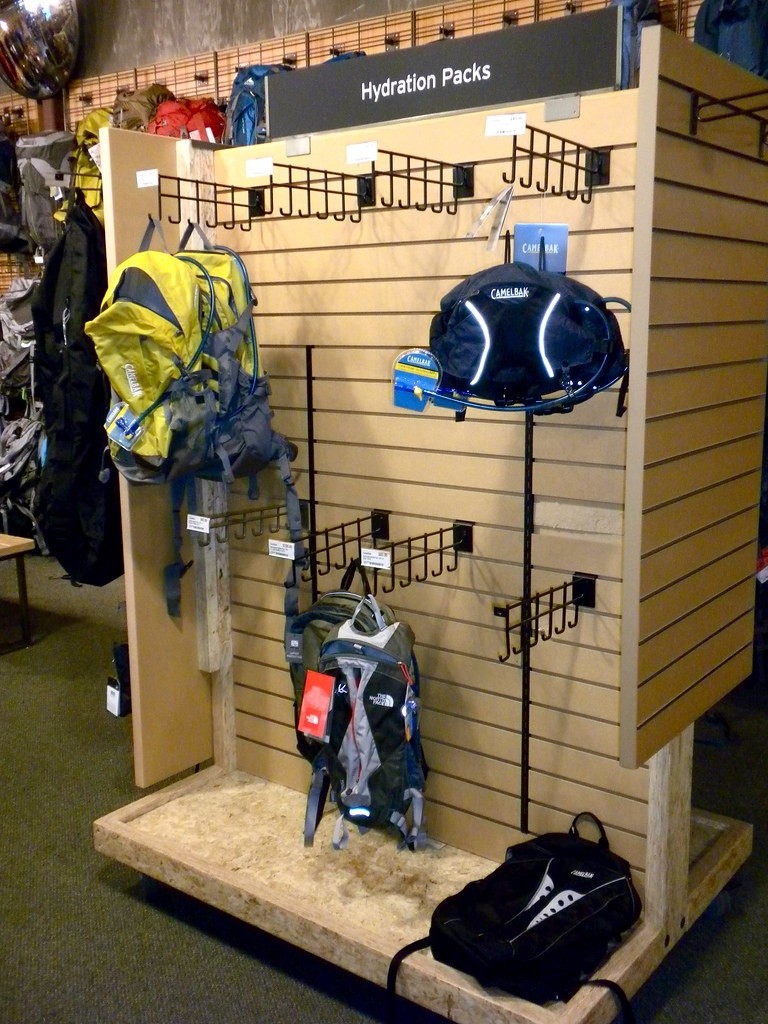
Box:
[428,230,630,422]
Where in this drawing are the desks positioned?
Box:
[0,535,35,646]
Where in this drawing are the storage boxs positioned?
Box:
[107,677,121,717]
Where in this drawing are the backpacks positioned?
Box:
[386,811,642,1024]
[604,0,768,91]
[0,189,125,588]
[0,50,368,259]
[285,557,447,853]
[83,217,305,618]
